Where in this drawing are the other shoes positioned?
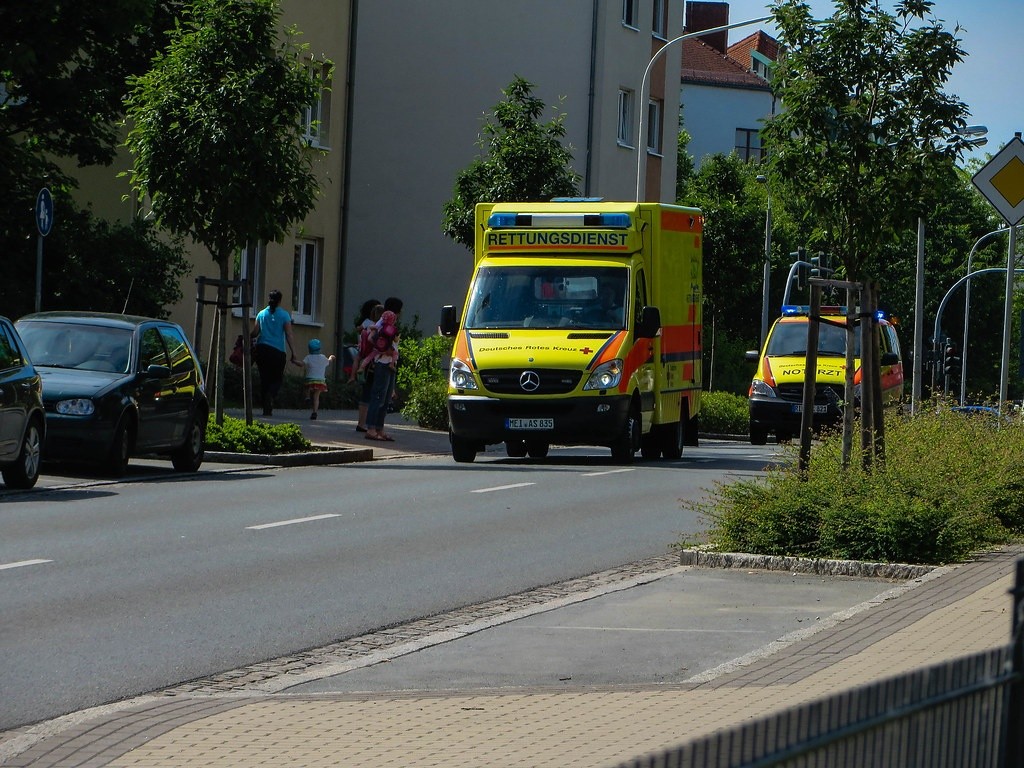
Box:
[377,430,395,441]
[303,399,312,407]
[389,364,396,372]
[347,378,358,386]
[365,432,386,441]
[263,408,272,416]
[357,369,364,375]
[310,413,317,420]
[356,426,367,433]
[391,393,397,400]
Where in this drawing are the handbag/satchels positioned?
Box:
[228,336,255,370]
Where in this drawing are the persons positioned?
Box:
[580,283,624,323]
[347,297,403,441]
[292,339,337,419]
[250,290,298,416]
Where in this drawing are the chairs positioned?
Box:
[498,285,535,320]
[109,346,129,372]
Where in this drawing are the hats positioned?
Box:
[381,311,396,326]
[308,339,322,354]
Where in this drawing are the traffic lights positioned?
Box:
[944,344,960,376]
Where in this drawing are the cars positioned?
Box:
[0,317,47,488]
[14,310,210,478]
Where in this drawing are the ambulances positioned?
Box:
[441,198,704,462]
[748,305,905,446]
